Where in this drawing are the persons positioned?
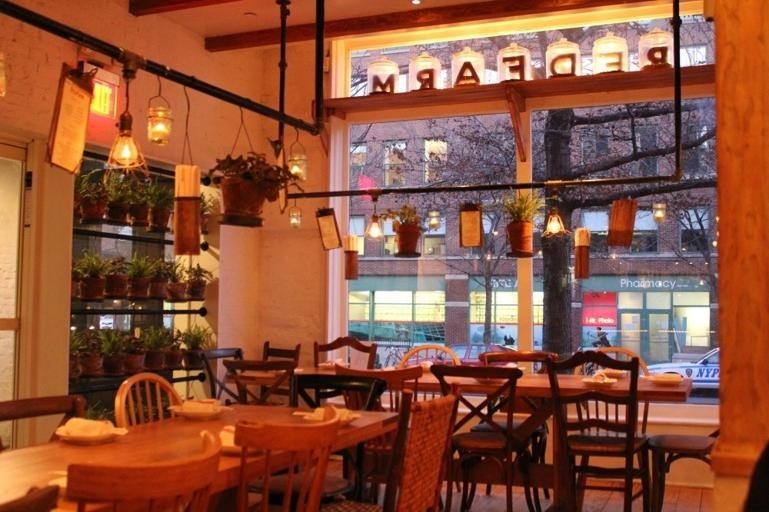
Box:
[586,325,613,348]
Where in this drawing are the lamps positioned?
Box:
[363,25,675,98]
[312,188,669,282]
[651,199,668,223]
[107,63,308,185]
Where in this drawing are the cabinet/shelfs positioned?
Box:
[69,151,209,395]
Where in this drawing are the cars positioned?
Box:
[646,346,720,393]
[400,343,538,374]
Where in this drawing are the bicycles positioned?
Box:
[382,344,423,367]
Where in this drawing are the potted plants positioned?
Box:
[68,323,214,381]
[200,150,305,229]
[75,170,215,236]
[71,247,213,302]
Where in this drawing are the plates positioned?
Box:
[167,406,233,420]
[54,426,129,443]
[49,476,70,498]
[291,411,361,427]
[604,372,625,379]
[582,379,618,389]
[646,377,685,385]
[219,446,262,456]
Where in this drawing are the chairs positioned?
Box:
[1,335,718,511]
